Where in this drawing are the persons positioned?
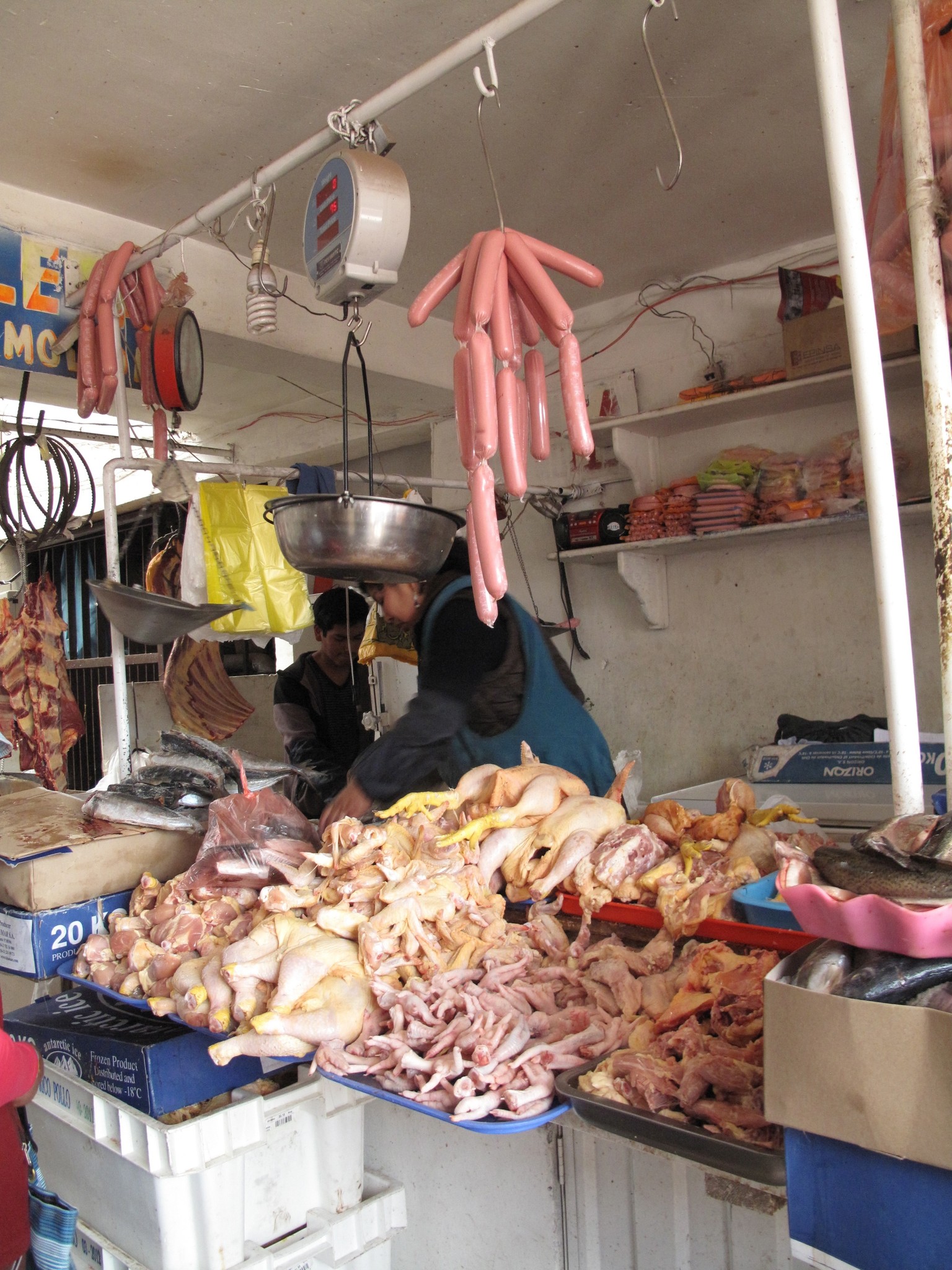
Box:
[274,586,371,815]
[317,537,629,831]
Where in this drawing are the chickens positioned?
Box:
[68,735,839,1177]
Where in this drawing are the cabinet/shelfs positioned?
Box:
[540,351,934,631]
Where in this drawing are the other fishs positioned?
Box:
[794,935,952,1007]
[80,721,332,835]
[812,844,952,907]
[851,811,952,875]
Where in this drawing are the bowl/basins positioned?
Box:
[266,494,468,585]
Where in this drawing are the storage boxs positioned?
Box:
[781,300,920,380]
[0,835,950,1269]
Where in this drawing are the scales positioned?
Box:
[265,145,468,589]
[85,305,253,644]
